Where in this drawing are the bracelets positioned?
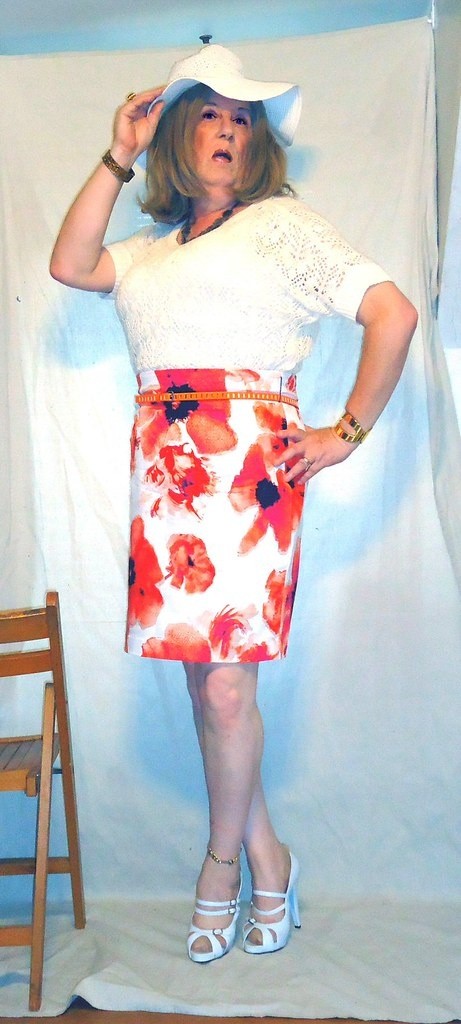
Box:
[102,150,135,182]
[335,408,373,445]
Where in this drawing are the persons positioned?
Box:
[49,44,419,964]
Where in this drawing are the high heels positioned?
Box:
[190,865,243,962]
[243,848,302,952]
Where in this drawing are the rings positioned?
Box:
[128,93,136,99]
[302,457,311,470]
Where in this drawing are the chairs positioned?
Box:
[0,590,86,1012]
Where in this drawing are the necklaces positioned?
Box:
[182,200,239,244]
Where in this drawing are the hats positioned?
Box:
[149,46,303,148]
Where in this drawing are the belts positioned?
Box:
[134,391,300,409]
